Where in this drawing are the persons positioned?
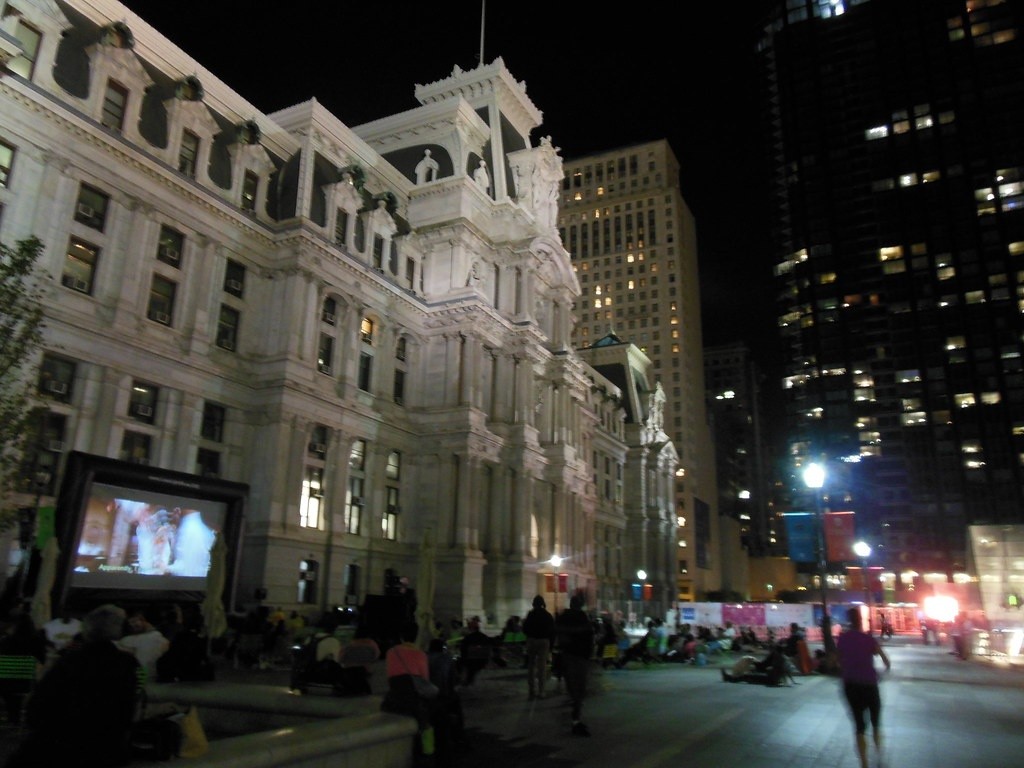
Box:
[877,614,892,639]
[0,575,826,768]
[837,608,891,768]
[948,611,990,659]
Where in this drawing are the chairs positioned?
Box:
[603,636,670,672]
[715,638,735,668]
[0,655,37,681]
[502,631,526,644]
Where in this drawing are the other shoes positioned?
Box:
[570,723,591,738]
[721,667,729,681]
[527,692,534,702]
[539,691,547,699]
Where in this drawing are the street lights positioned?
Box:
[550,555,562,617]
[854,541,870,634]
[637,568,648,621]
[802,462,836,654]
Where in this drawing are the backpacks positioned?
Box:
[291,634,329,689]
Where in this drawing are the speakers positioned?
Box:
[254,588,267,600]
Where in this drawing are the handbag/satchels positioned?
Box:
[414,675,441,702]
[167,703,211,758]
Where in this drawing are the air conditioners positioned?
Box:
[133,404,153,417]
[44,380,68,395]
[397,351,405,360]
[362,333,372,342]
[324,313,336,323]
[205,472,217,478]
[354,496,365,506]
[152,312,170,324]
[311,442,326,453]
[389,504,402,514]
[227,279,241,291]
[76,203,95,221]
[409,289,416,295]
[161,248,178,260]
[43,439,64,453]
[312,489,325,497]
[395,397,403,406]
[137,458,152,466]
[219,339,233,350]
[320,365,331,374]
[339,244,349,251]
[376,268,385,275]
[66,276,89,293]
